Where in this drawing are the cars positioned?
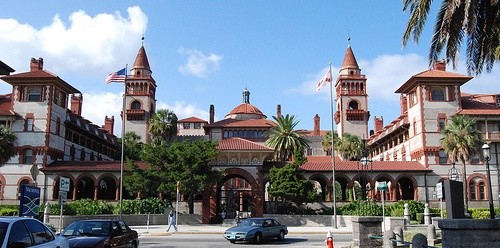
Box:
[223,217,288,244]
[0,216,70,248]
[56,216,140,248]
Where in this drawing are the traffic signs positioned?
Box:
[59,176,70,192]
[376,180,388,191]
[58,190,67,205]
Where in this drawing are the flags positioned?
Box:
[106,68,126,85]
[316,70,331,93]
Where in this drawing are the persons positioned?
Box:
[166,210,177,232]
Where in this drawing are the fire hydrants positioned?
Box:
[324,230,334,248]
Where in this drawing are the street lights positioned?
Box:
[447,161,461,181]
[357,138,373,216]
[481,142,495,219]
